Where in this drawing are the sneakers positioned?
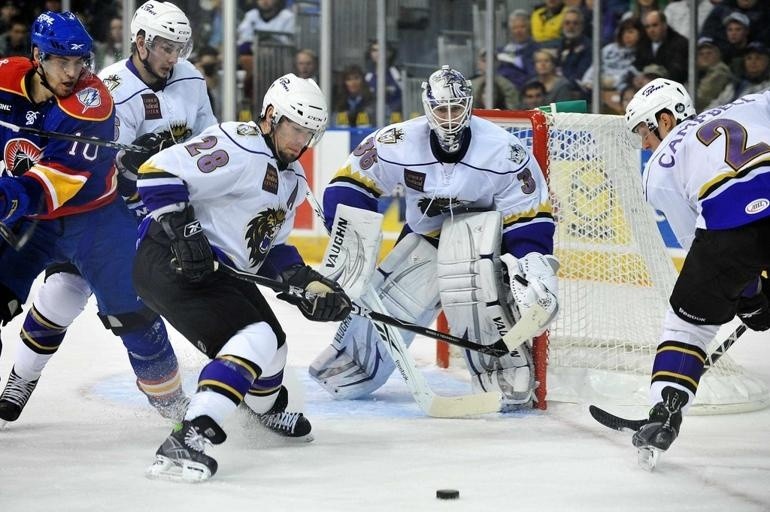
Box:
[158,394,191,420]
[0,365,40,422]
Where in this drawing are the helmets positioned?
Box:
[422,65,475,154]
[260,73,329,150]
[625,77,695,151]
[130,1,194,62]
[29,10,94,81]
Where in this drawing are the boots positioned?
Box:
[156,416,227,474]
[632,386,689,450]
[261,385,312,438]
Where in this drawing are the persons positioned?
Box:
[1,1,405,126]
[460,0,770,114]
[625,77,770,472]
[131,72,353,481]
[309,65,560,411]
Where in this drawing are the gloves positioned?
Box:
[735,275,770,332]
[119,131,173,174]
[162,205,217,289]
[277,265,352,323]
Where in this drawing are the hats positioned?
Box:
[696,36,718,49]
[723,10,751,28]
[743,40,766,54]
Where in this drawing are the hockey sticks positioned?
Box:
[303,188,499,419]
[588,323,747,434]
[170,257,545,357]
[1,121,151,153]
